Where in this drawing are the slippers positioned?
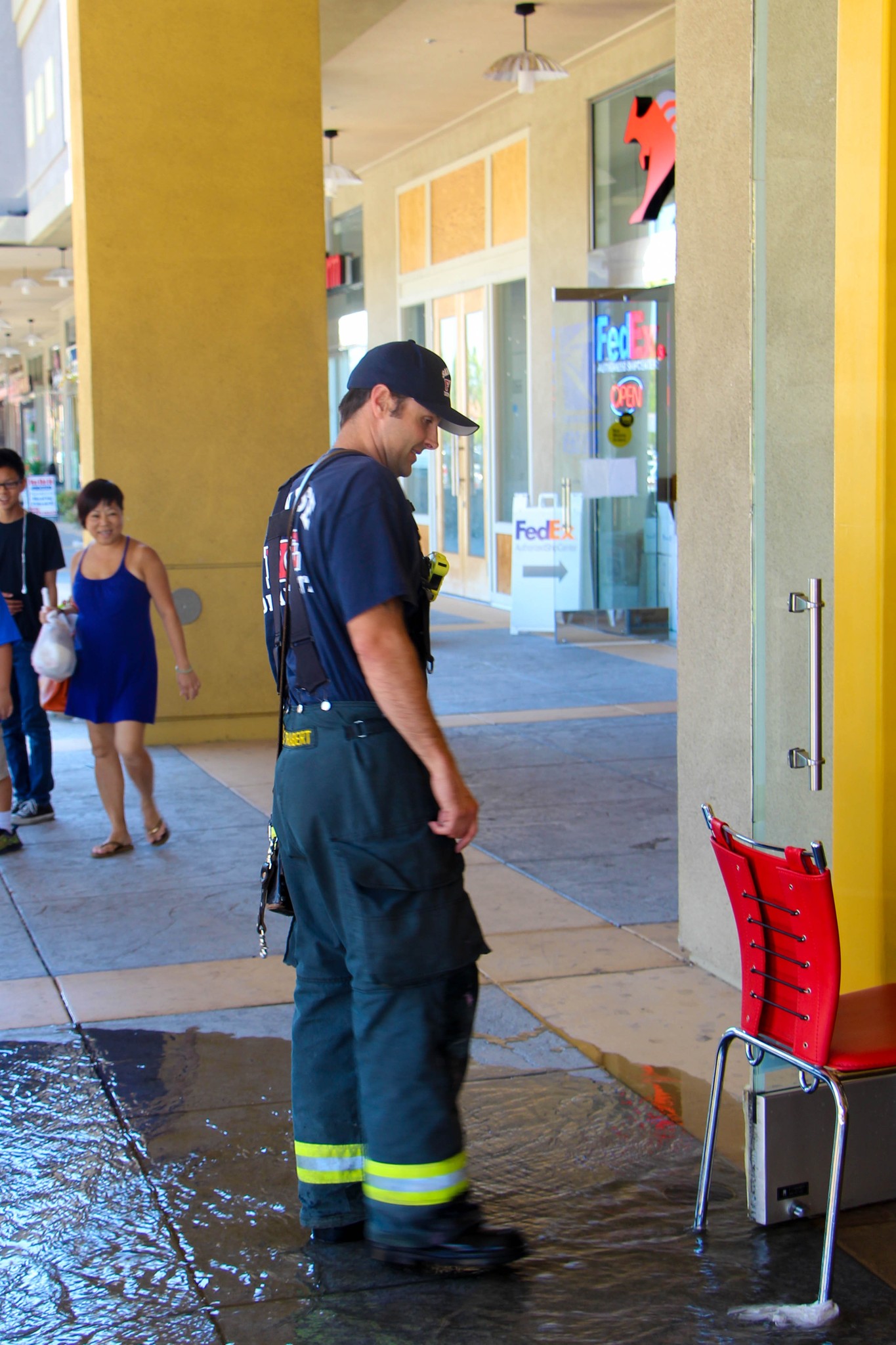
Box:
[90,839,135,859]
[144,816,170,848]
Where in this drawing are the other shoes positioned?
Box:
[371,1220,532,1274]
[310,1219,370,1244]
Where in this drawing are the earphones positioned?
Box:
[21,585,27,594]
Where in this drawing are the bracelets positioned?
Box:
[173,665,195,674]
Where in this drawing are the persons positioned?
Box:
[260,342,532,1273]
[38,479,202,858]
[0,447,67,856]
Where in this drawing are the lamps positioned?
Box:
[483,3,571,95]
[323,129,362,200]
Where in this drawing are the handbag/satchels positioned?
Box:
[30,610,80,680]
[37,599,82,713]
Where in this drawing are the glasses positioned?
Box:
[0,478,21,491]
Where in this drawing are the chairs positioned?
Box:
[688,805,895,1316]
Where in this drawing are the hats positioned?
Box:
[346,339,481,437]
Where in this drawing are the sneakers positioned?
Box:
[10,799,27,815]
[0,822,24,855]
[10,798,56,826]
